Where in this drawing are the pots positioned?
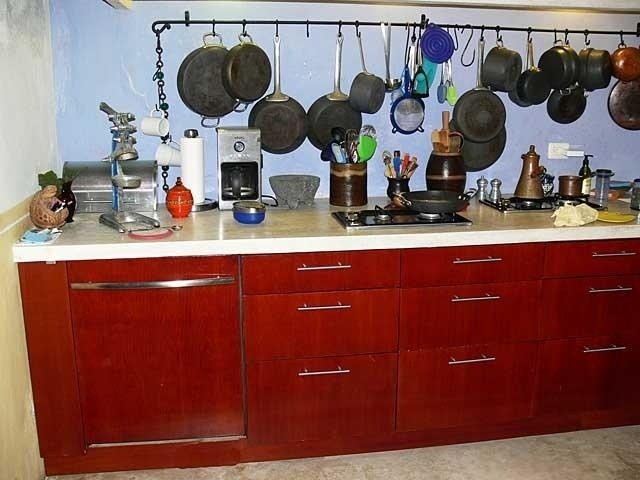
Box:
[349,26,426,136]
[309,28,362,150]
[395,190,469,212]
[559,176,584,196]
[178,32,237,128]
[447,27,638,144]
[248,34,309,154]
[223,32,270,114]
[448,40,506,173]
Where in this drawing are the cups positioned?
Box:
[156,140,182,166]
[142,108,171,137]
[330,162,368,206]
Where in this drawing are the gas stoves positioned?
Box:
[478,193,606,212]
[334,207,475,228]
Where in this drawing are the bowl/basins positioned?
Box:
[232,202,265,224]
[268,175,320,209]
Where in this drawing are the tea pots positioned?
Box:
[513,145,545,200]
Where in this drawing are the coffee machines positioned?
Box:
[216,127,261,212]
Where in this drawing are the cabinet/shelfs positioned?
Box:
[547,239,640,418]
[64,253,247,451]
[399,242,546,435]
[237,249,400,446]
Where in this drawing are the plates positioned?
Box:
[610,180,631,191]
[595,210,635,223]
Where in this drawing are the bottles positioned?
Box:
[386,178,410,201]
[180,130,205,205]
[166,176,195,219]
[595,168,614,202]
[630,178,640,210]
[476,175,502,201]
